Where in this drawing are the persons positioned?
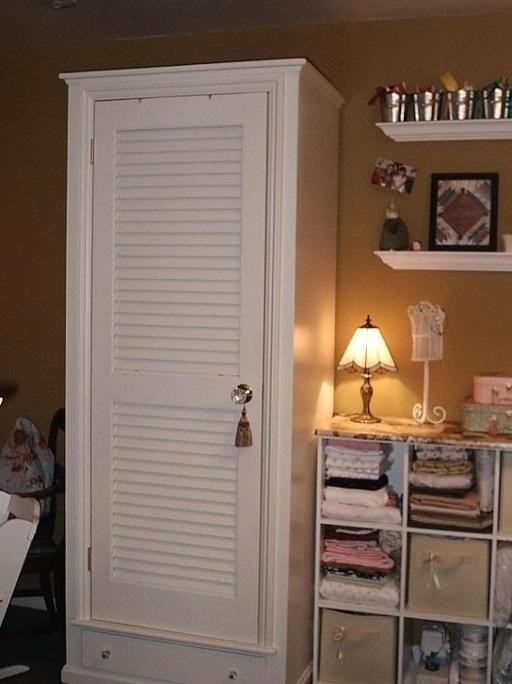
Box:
[405,168,415,193]
[393,167,407,192]
[374,164,393,188]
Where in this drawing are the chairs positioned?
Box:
[7,407,66,636]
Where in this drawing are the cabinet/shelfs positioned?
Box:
[311,418,510,682]
[373,120,510,273]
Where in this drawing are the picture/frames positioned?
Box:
[425,171,500,252]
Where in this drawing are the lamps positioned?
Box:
[337,312,400,422]
